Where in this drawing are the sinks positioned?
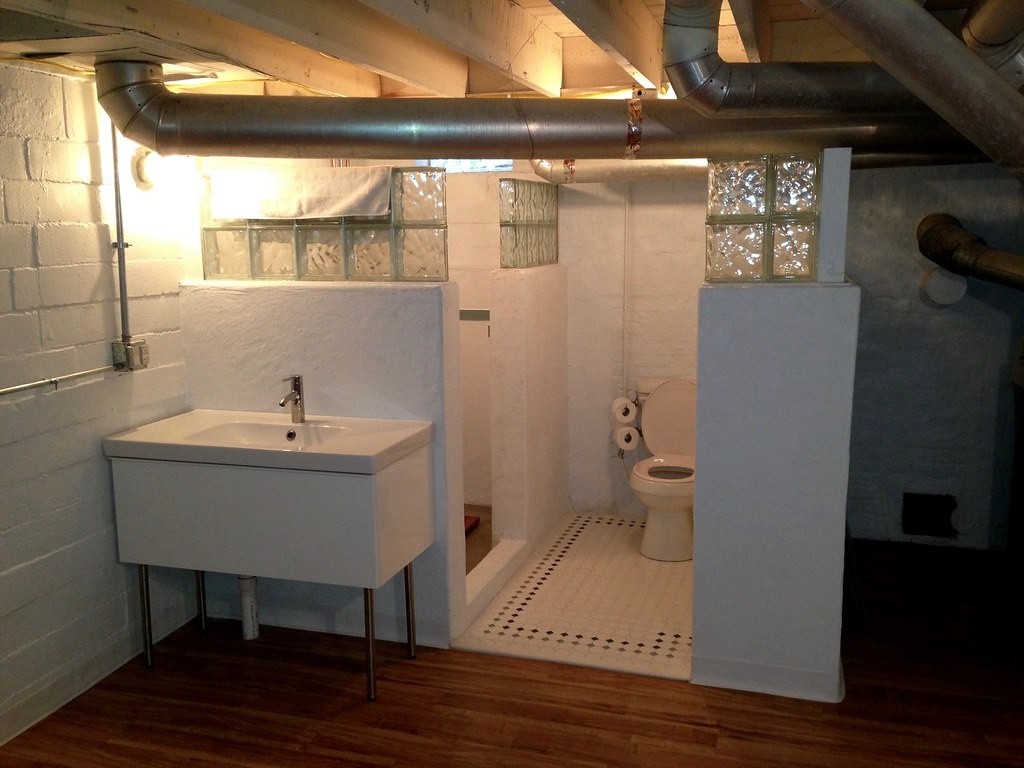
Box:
[103,409,434,476]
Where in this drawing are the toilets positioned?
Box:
[630,379,698,562]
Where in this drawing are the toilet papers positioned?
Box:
[611,396,640,451]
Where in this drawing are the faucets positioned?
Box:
[279,374,306,423]
